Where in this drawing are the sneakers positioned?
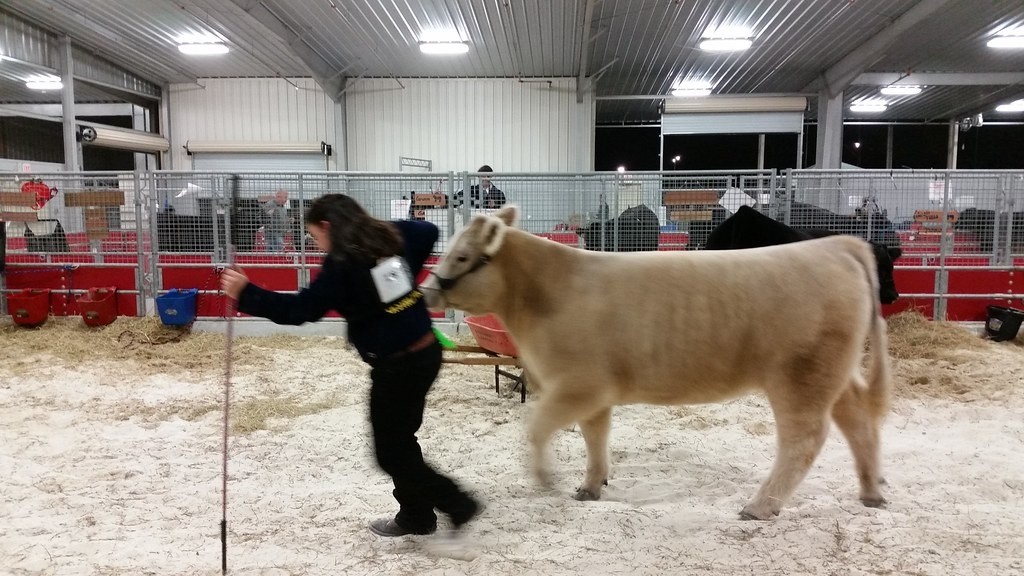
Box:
[468,496,486,516]
[370,518,438,537]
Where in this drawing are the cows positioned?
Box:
[767,200,902,248]
[576,203,661,252]
[417,205,892,522]
[701,205,904,306]
[951,207,1024,255]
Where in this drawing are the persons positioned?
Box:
[262,188,293,252]
[220,194,484,538]
[595,192,611,220]
[453,164,505,208]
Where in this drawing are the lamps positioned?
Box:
[987,34,1024,49]
[848,104,890,113]
[877,87,922,95]
[699,37,754,52]
[418,40,472,55]
[24,82,65,90]
[178,42,231,56]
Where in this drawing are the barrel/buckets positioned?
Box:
[155,290,197,325]
[7,287,50,326]
[76,287,118,327]
[985,305,1024,339]
